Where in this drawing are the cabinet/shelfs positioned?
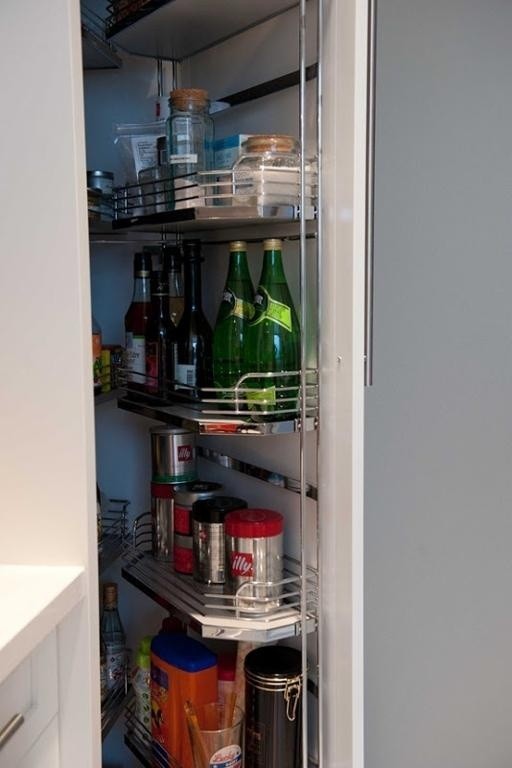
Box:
[116,184,319,766]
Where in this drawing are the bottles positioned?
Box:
[224,508,284,616]
[129,634,152,749]
[87,169,115,221]
[185,702,244,768]
[243,239,299,420]
[149,423,198,485]
[151,480,225,574]
[167,87,216,209]
[192,495,247,595]
[138,166,168,215]
[98,581,126,706]
[212,240,256,416]
[234,134,312,206]
[244,645,303,768]
[124,240,208,404]
